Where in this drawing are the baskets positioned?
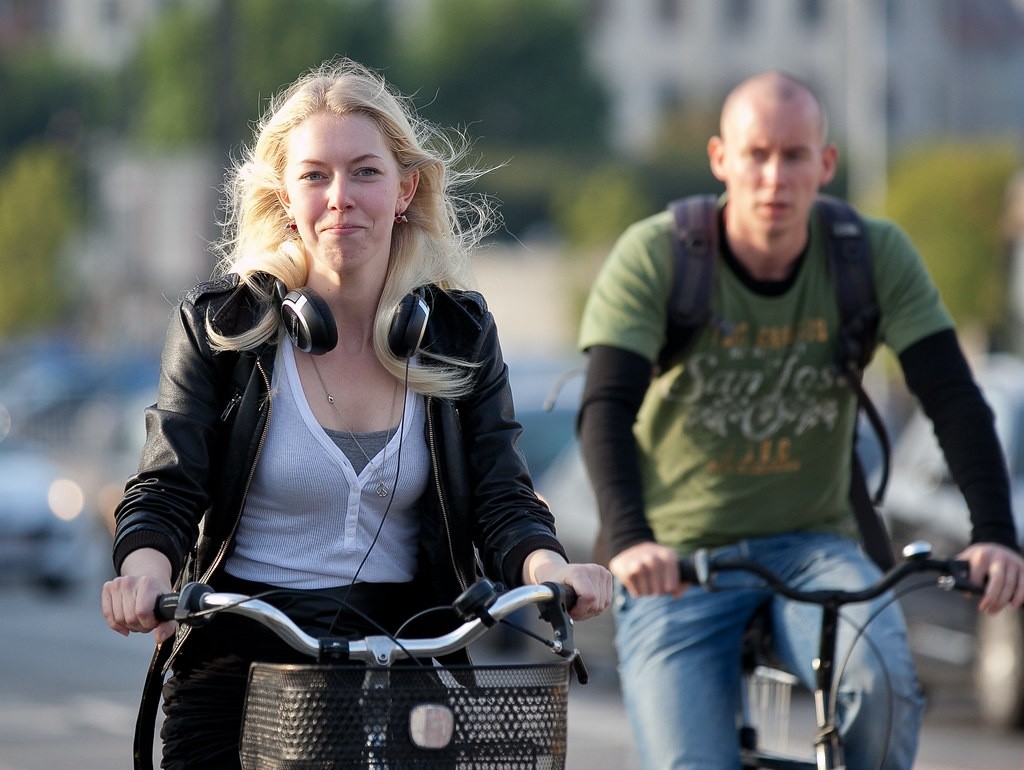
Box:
[234,661,572,770]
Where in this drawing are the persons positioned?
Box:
[101,56,613,770]
[577,73,1024,770]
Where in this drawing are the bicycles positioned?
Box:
[151,576,590,770]
[615,540,1016,770]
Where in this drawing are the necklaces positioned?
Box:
[310,354,398,498]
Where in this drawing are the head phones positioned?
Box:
[275,278,430,358]
